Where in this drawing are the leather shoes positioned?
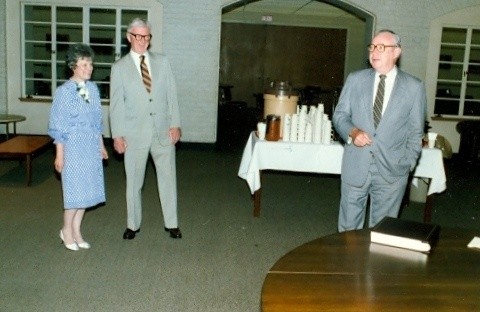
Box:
[123,228,140,240]
[164,227,182,238]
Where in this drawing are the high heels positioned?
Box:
[73,238,90,249]
[59,229,80,251]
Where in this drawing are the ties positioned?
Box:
[373,74,387,130]
[139,55,151,93]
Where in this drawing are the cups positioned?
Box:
[282,103,335,143]
[265,115,280,141]
[427,132,438,149]
[257,123,266,139]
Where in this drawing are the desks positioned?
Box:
[260,223,480,312]
[0,135,54,187]
[0,115,26,140]
[235,129,448,226]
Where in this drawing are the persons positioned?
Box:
[110,17,183,240]
[332,29,427,233]
[47,44,109,251]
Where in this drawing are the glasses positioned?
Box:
[367,43,397,53]
[129,32,152,41]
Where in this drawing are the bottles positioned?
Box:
[263,81,299,138]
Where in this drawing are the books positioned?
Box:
[369,216,441,253]
